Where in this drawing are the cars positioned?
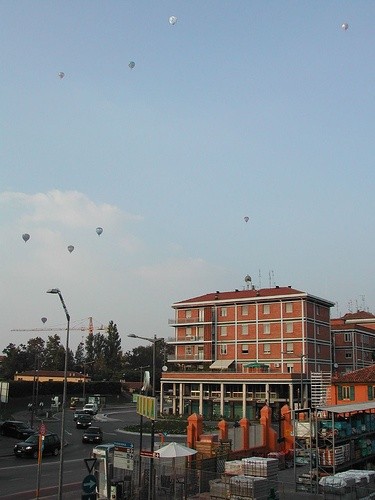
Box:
[82,426,103,443]
[1,420,35,439]
[73,410,86,421]
[90,444,114,463]
[13,431,61,457]
[74,414,92,427]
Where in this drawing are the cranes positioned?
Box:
[10,316,107,340]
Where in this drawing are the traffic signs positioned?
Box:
[113,441,134,471]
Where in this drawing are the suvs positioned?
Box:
[83,403,97,415]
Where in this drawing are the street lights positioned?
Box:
[46,287,71,500]
[127,332,164,470]
[279,353,310,408]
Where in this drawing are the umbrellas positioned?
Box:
[154,442,198,467]
